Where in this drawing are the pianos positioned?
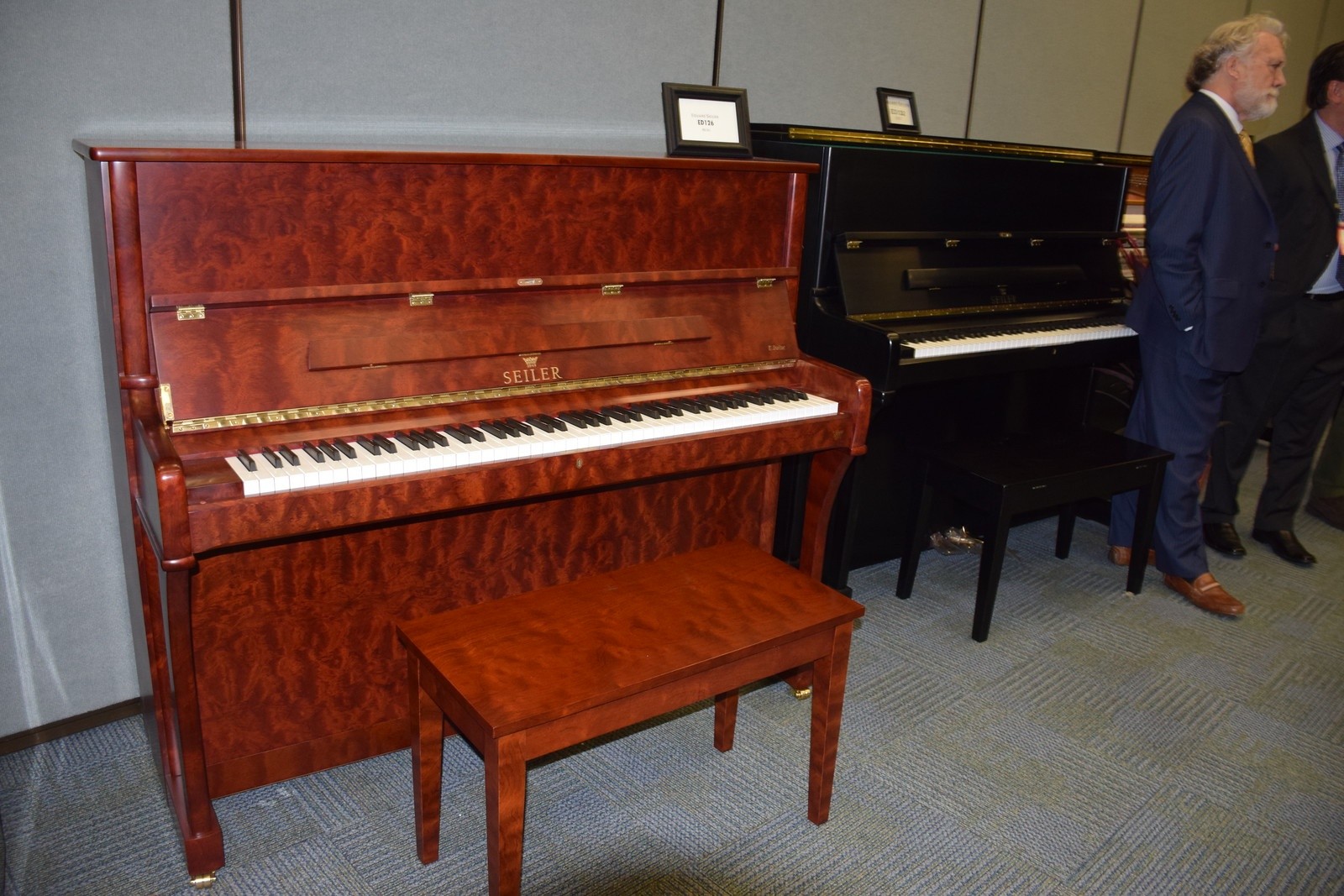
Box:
[747,117,1140,597]
[71,138,873,889]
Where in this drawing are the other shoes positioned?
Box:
[1306,497,1344,530]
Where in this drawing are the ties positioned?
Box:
[1334,141,1344,287]
[1239,130,1256,169]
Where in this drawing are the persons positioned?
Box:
[1109,12,1291,619]
[1201,41,1344,568]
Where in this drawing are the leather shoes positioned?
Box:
[1251,522,1319,568]
[1109,544,1157,566]
[1204,522,1247,560]
[1165,569,1247,617]
